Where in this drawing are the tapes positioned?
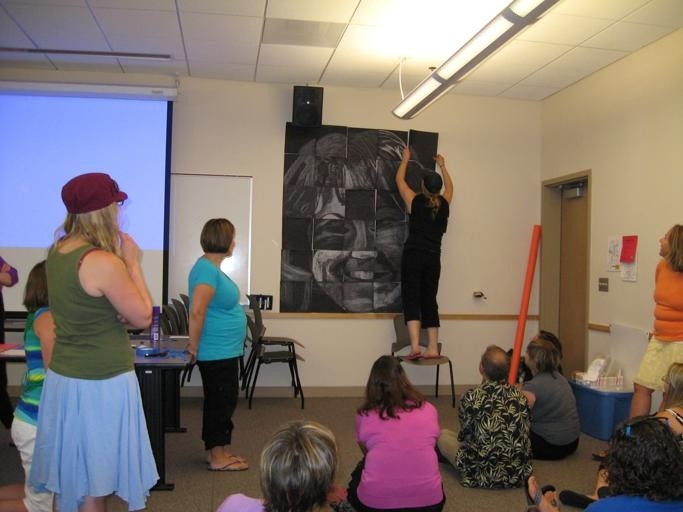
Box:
[136,347,160,356]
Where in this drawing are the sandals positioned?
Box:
[406,351,424,360]
[422,352,441,359]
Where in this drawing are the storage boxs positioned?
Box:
[569,378,632,442]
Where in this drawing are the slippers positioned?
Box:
[592,449,609,461]
[540,484,560,512]
[524,476,543,507]
[205,461,249,472]
[205,451,247,464]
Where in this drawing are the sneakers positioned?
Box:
[559,490,600,510]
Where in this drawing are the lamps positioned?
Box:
[392,0,560,120]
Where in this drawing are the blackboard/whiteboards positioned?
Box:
[165,173,251,307]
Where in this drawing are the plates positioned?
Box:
[127,329,144,335]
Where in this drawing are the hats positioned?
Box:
[422,166,443,193]
[60,172,128,215]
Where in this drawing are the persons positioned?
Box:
[216,419,339,512]
[27,171,161,512]
[11,259,56,512]
[283,130,419,313]
[524,331,562,382]
[524,414,683,512]
[184,217,249,470]
[344,354,446,512]
[394,148,454,360]
[0,258,19,446]
[438,347,532,490]
[517,338,581,461]
[559,363,683,508]
[592,223,683,459]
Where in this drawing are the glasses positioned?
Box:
[112,200,124,209]
[661,375,670,385]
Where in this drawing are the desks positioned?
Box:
[1,337,186,491]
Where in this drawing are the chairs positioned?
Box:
[160,293,305,409]
[391,313,456,406]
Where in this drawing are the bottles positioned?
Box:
[256,294,263,309]
[149,306,161,351]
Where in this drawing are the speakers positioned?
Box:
[291,86,323,129]
